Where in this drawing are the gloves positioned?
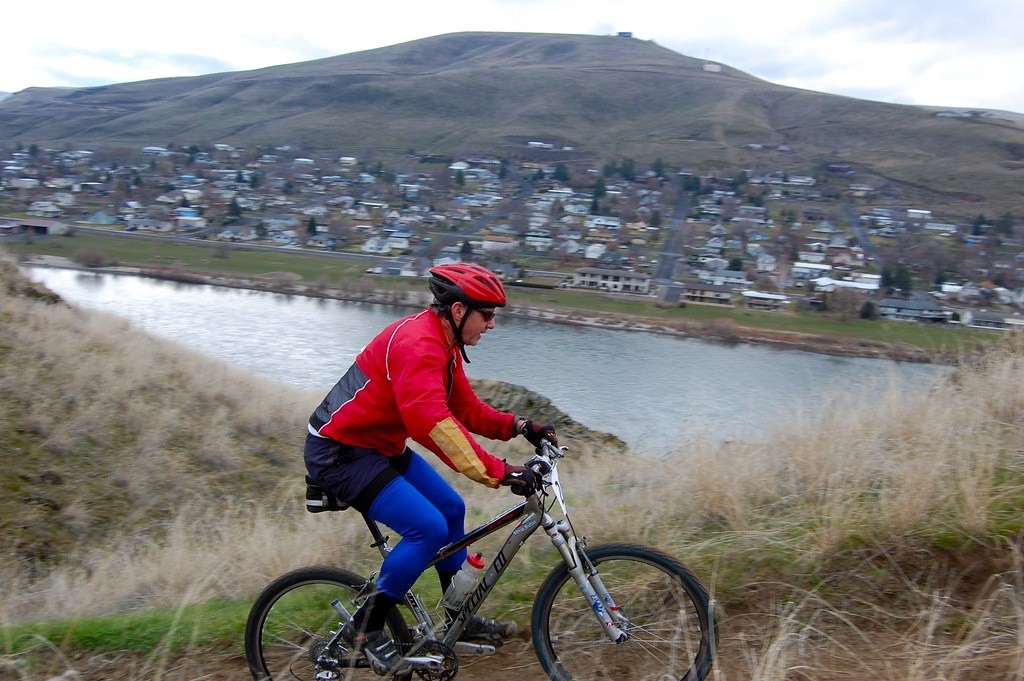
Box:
[500,463,543,496]
[519,420,558,455]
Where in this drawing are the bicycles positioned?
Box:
[245,438,721,681]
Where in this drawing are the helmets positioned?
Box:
[428,263,507,308]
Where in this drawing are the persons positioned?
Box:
[304,262,558,677]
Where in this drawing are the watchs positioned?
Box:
[513,416,525,438]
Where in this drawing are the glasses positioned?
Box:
[476,308,496,322]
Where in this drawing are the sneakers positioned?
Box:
[441,615,518,642]
[342,617,413,675]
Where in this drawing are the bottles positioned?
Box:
[444,550,486,610]
